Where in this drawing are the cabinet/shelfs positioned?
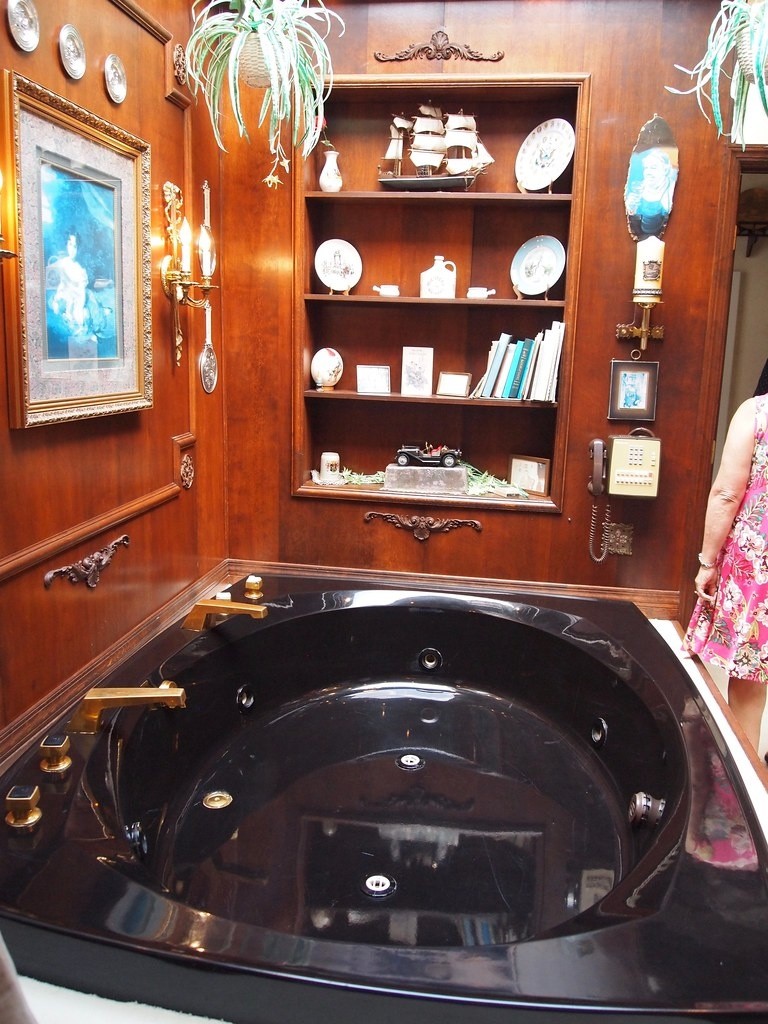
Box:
[287,73,592,517]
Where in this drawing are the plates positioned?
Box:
[509,236,565,295]
[315,239,362,290]
[514,119,575,190]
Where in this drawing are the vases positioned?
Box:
[319,152,343,192]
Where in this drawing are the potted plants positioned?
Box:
[182,1,347,189]
[663,1,768,151]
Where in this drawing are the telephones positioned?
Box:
[587,435,661,498]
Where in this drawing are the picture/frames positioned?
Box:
[506,454,550,497]
[436,372,472,397]
[3,68,157,429]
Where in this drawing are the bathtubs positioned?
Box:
[106,588,696,948]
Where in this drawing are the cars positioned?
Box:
[394,441,463,467]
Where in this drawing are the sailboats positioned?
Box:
[376,97,495,191]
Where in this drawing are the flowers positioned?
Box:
[313,115,336,150]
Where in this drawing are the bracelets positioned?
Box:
[698,552,717,568]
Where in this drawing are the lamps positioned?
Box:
[160,179,218,364]
[616,234,667,351]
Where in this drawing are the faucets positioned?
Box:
[65,687,187,733]
[180,599,268,632]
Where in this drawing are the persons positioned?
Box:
[681,354,768,756]
[47,230,87,334]
[682,692,760,874]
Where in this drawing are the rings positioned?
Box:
[694,590,700,597]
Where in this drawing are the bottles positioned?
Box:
[419,255,458,300]
[319,150,342,193]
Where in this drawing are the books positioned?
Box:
[469,320,566,405]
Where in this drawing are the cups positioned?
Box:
[466,286,496,299]
[320,452,340,483]
[374,285,400,296]
